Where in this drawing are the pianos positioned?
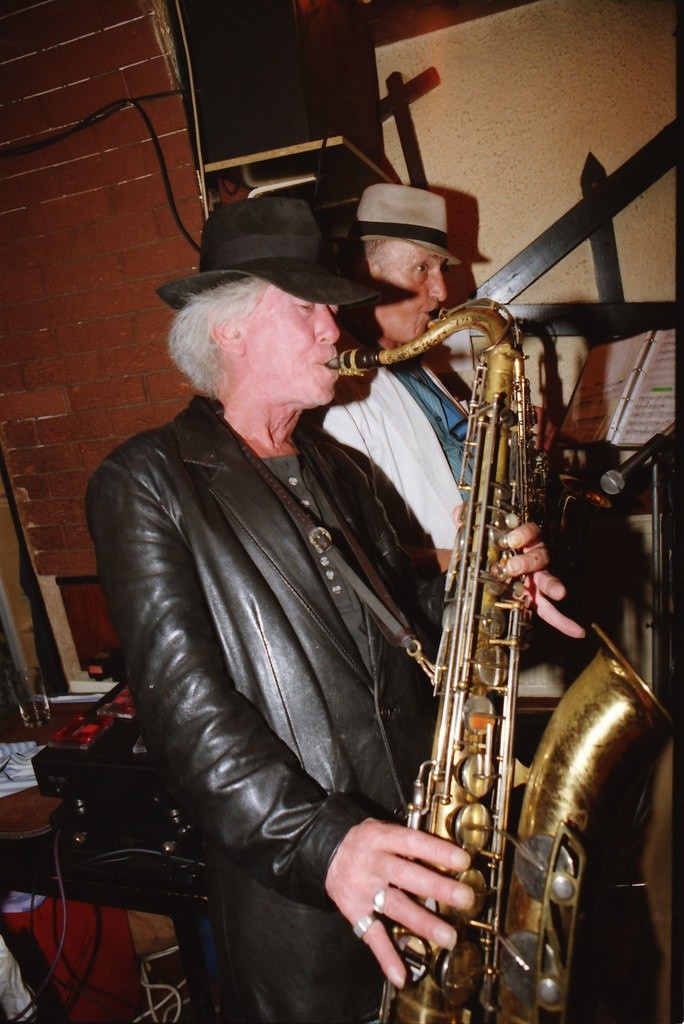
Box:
[3,678,222,1024]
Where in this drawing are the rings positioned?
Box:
[375,887,387,912]
[350,916,375,938]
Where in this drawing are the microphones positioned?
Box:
[601,423,675,495]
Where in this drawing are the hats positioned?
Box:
[327,183,461,265]
[156,195,383,308]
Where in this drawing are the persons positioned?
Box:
[319,183,472,583]
[85,194,587,1024]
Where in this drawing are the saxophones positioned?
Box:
[321,293,681,1024]
[427,307,615,660]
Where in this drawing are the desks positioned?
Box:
[0,831,236,1024]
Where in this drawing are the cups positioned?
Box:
[7,666,51,728]
